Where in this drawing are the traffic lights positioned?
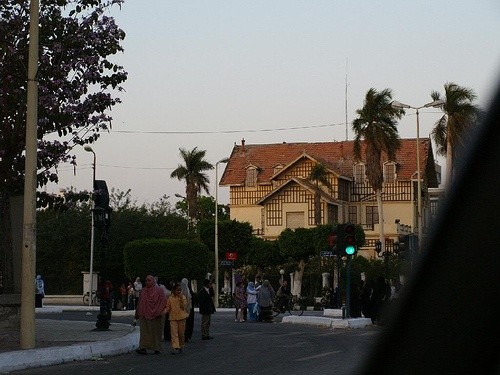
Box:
[328,224,342,255]
[342,223,357,256]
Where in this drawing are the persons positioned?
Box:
[131,275,167,354]
[276,280,288,298]
[119,282,127,310]
[235,273,275,322]
[154,277,172,315]
[195,279,216,339]
[35,275,45,307]
[127,281,136,310]
[133,277,143,310]
[103,280,113,308]
[160,284,189,351]
[181,278,196,343]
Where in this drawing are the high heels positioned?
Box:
[239,318,245,322]
[234,319,237,322]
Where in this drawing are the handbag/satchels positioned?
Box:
[257,306,261,316]
[253,302,258,313]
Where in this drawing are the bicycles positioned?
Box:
[272,295,304,317]
[82,290,100,306]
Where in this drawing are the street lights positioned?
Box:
[82,144,97,306]
[391,98,445,251]
[279,269,285,284]
[90,201,115,331]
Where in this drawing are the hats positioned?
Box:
[255,274,261,279]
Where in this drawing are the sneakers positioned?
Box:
[155,350,160,354]
[171,350,179,354]
[136,349,147,355]
[202,335,214,340]
[178,348,183,353]
